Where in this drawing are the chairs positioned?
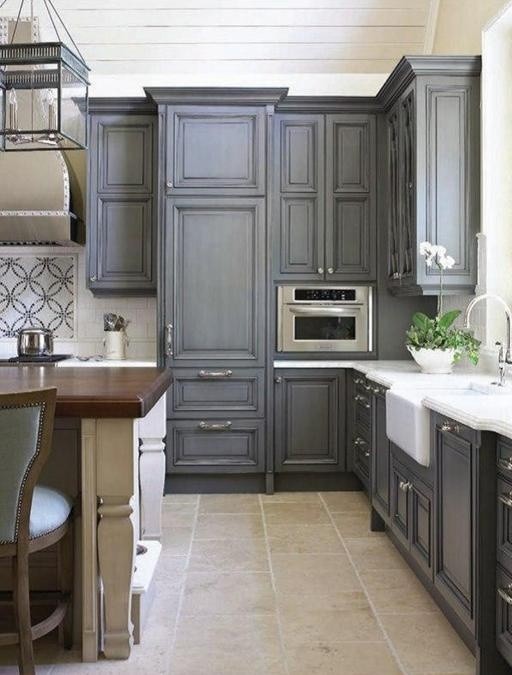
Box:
[0,386,78,675]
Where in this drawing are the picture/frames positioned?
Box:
[0,253,78,343]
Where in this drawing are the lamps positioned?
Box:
[0,0,90,153]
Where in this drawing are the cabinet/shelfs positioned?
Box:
[377,55,480,296]
[144,85,290,494]
[476,431,511,674]
[372,385,479,651]
[73,98,157,297]
[345,368,377,501]
[273,95,379,281]
[274,368,346,477]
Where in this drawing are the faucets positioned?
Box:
[462,292,512,387]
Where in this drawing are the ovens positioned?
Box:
[277,284,374,354]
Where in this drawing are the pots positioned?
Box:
[16,327,56,358]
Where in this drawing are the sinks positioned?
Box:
[387,371,496,466]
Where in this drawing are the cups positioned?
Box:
[104,331,126,360]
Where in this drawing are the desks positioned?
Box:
[0,367,174,662]
